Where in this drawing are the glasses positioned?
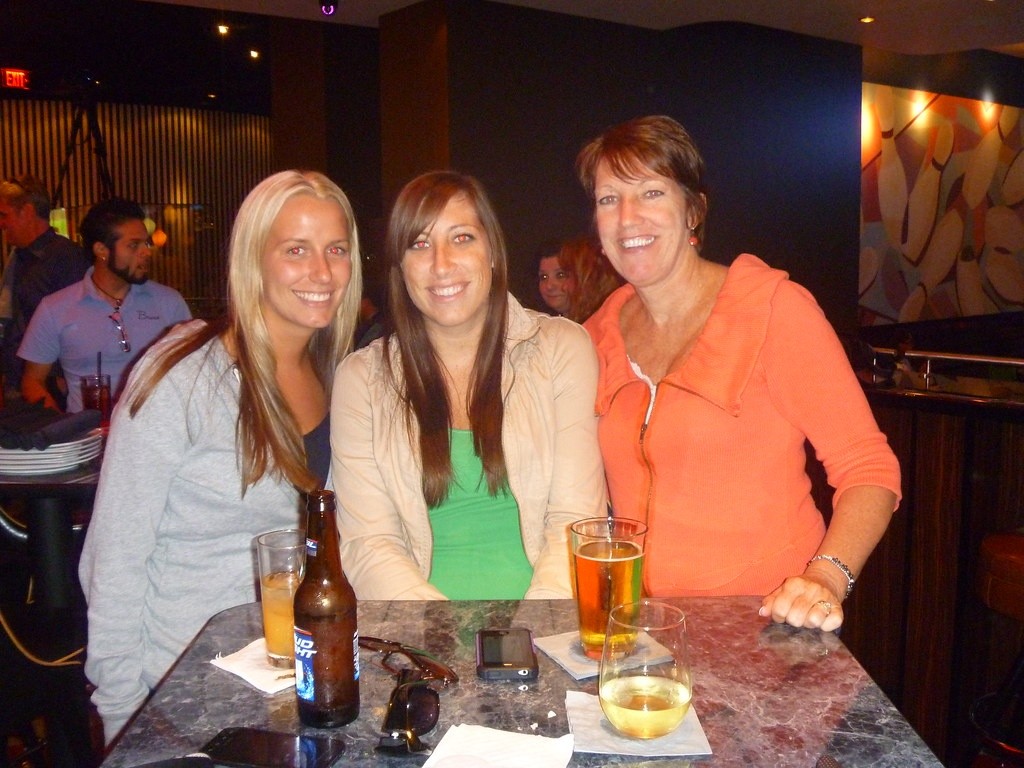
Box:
[376,675,440,751]
[109,309,131,353]
[356,631,456,684]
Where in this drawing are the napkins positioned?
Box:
[532,629,673,680]
[421,723,574,768]
[564,690,712,756]
[210,638,296,694]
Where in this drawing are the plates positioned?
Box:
[0,428,103,476]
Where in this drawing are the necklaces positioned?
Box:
[91,276,122,305]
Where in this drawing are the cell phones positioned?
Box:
[198,728,346,768]
[475,628,539,681]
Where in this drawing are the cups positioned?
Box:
[81,375,112,437]
[568,517,648,661]
[599,601,692,739]
[257,529,306,669]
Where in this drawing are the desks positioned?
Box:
[103,599,946,768]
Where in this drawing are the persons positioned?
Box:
[579,116,902,634]
[15,196,192,416]
[536,229,615,326]
[79,167,363,752]
[0,177,86,333]
[327,170,611,600]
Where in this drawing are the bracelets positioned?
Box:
[811,554,856,597]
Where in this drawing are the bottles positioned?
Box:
[293,491,359,729]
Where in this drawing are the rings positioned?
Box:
[817,600,832,615]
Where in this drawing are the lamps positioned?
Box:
[217,9,228,34]
